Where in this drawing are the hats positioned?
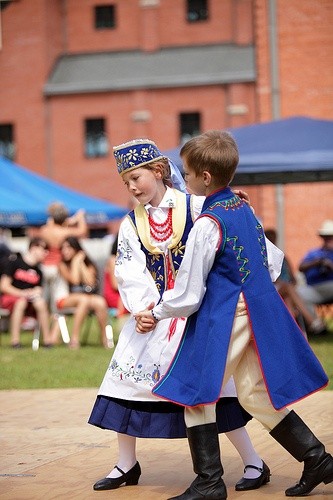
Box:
[318,220,333,235]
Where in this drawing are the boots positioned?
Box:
[166,421,227,500]
[269,409,333,497]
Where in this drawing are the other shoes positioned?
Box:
[235,459,271,491]
[93,461,142,491]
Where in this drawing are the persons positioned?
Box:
[264,221,333,341]
[88,139,284,492]
[132,131,333,500]
[0,202,131,350]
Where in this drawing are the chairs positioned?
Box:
[0,234,118,352]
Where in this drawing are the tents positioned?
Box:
[161,116,333,186]
[0,155,130,228]
